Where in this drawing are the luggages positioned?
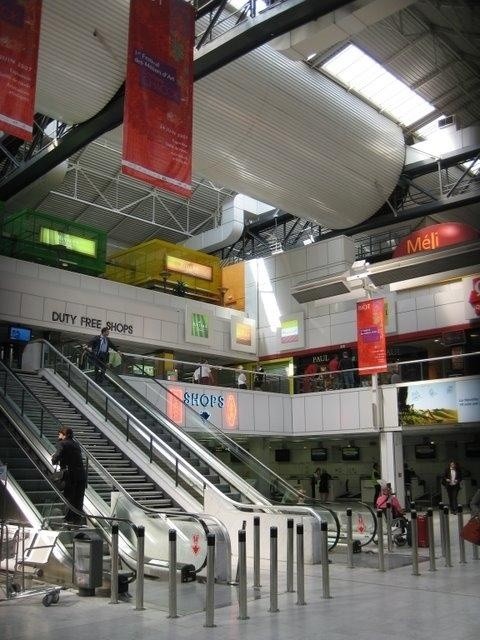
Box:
[407,515,430,548]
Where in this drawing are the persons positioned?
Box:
[200,360,215,386]
[51,426,88,538]
[233,350,404,393]
[318,467,332,503]
[81,327,122,384]
[310,467,321,498]
[404,461,413,513]
[193,360,201,382]
[442,458,463,515]
[376,487,410,535]
[469,487,480,521]
[372,462,382,508]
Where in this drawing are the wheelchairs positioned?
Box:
[372,508,409,547]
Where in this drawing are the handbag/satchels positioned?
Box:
[48,464,67,490]
[460,517,480,546]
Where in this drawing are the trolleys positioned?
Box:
[0,523,66,607]
